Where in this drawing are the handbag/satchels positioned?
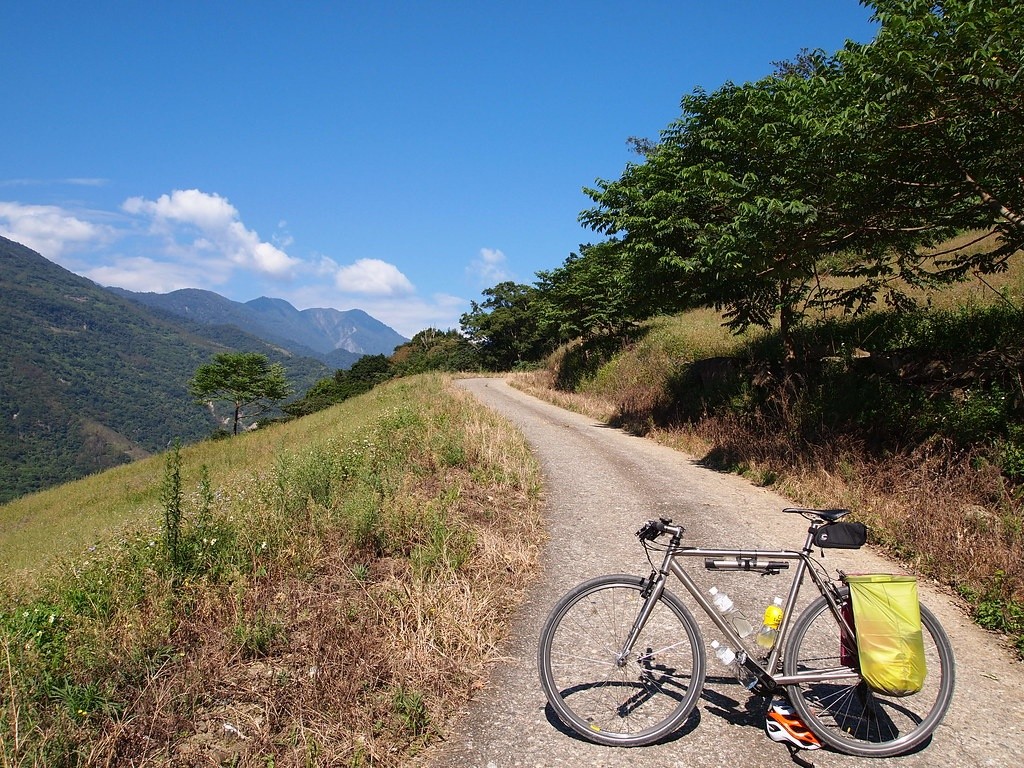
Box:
[843,573,926,697]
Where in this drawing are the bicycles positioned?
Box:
[537,506,957,759]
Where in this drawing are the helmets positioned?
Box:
[767,696,826,750]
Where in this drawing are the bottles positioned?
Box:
[757,597,785,648]
[710,640,759,690]
[709,586,753,638]
